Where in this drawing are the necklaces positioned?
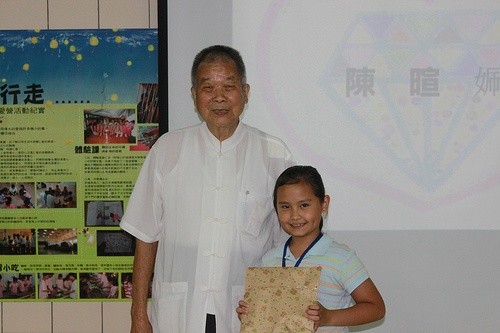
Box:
[281,232,324,266]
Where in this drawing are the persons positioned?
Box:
[0,90,160,301]
[118,44,296,333]
[236,165,386,332]
